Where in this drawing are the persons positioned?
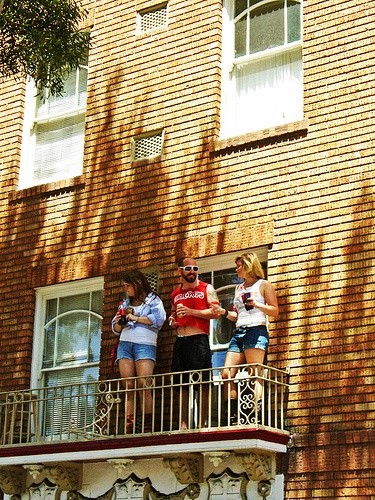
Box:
[111,269,166,433]
[208,252,279,426]
[165,257,221,430]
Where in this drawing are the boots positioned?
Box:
[225,397,239,426]
[144,414,153,432]
[251,399,262,425]
[126,414,134,434]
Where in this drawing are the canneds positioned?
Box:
[176,303,184,318]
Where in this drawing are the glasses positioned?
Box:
[180,265,199,272]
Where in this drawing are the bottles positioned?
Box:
[117,301,127,325]
[240,286,254,311]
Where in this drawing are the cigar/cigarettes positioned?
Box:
[169,319,172,326]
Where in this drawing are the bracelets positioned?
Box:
[137,317,139,322]
[221,309,228,318]
